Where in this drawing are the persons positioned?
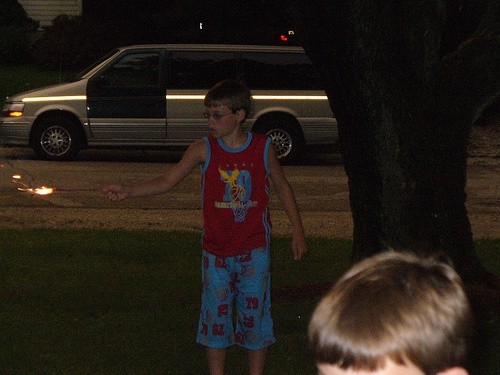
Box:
[307,248,473,375]
[97,76,307,375]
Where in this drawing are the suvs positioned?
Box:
[0,43,340,166]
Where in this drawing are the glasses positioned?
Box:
[202,112,234,121]
